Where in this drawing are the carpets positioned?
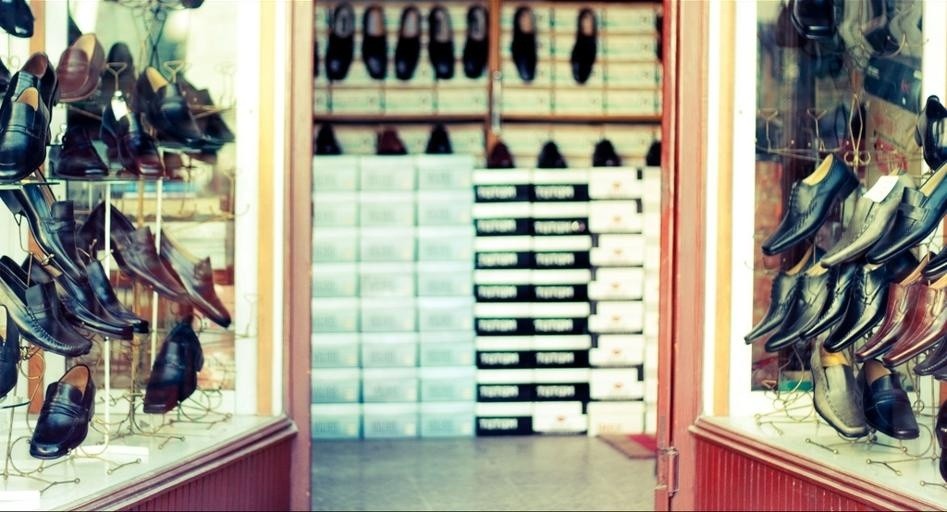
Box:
[598,433,656,458]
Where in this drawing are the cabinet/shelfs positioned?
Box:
[2,417,298,512]
[0,60,234,498]
[755,0,947,494]
[687,419,947,512]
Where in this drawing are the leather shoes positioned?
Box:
[762,153,860,256]
[75,246,149,335]
[0,164,90,287]
[313,125,342,155]
[324,0,356,81]
[48,125,110,180]
[131,66,210,149]
[462,4,490,80]
[788,0,839,41]
[799,256,857,340]
[53,32,105,103]
[934,366,947,381]
[569,8,598,85]
[854,251,938,362]
[912,335,947,376]
[809,338,867,438]
[30,364,96,461]
[0,86,52,184]
[823,244,919,353]
[99,90,163,178]
[425,122,452,153]
[0,304,20,400]
[142,323,204,414]
[537,142,567,168]
[21,254,134,341]
[511,5,538,82]
[375,129,407,155]
[151,228,231,328]
[744,243,825,346]
[1,255,93,358]
[593,139,621,166]
[864,162,947,265]
[764,244,839,353]
[0,51,60,147]
[394,5,422,80]
[883,245,947,368]
[646,141,661,166]
[914,95,947,170]
[0,0,34,39]
[852,358,919,440]
[165,69,236,142]
[860,0,902,53]
[486,143,515,169]
[78,198,190,305]
[96,40,138,135]
[426,4,456,80]
[820,169,916,269]
[361,5,389,80]
